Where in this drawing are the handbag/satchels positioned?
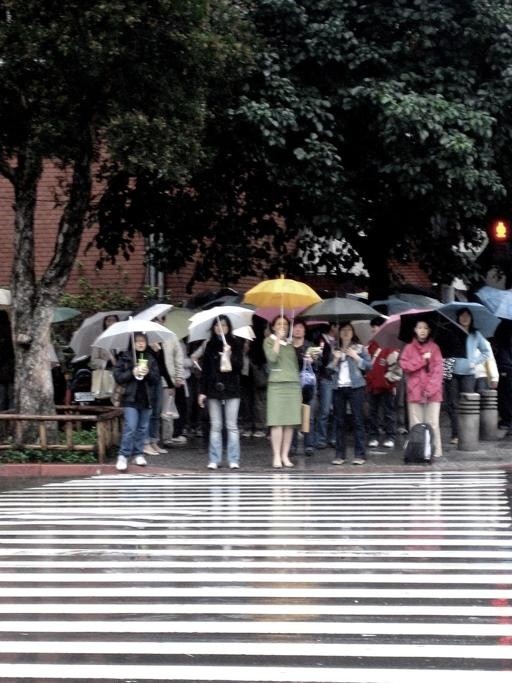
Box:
[300,370,318,387]
[299,403,310,433]
[91,370,116,399]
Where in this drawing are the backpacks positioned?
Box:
[401,423,434,466]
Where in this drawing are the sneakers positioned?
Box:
[382,440,394,448]
[450,436,458,444]
[367,439,378,446]
[289,436,336,455]
[130,455,147,466]
[115,455,129,470]
[331,458,345,464]
[238,428,266,438]
[228,462,239,468]
[205,462,218,469]
[143,425,203,456]
[351,458,366,466]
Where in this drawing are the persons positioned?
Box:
[1,308,511,470]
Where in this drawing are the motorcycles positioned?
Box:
[69,353,101,429]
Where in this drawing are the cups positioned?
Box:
[137,358,149,377]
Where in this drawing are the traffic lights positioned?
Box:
[490,218,512,242]
